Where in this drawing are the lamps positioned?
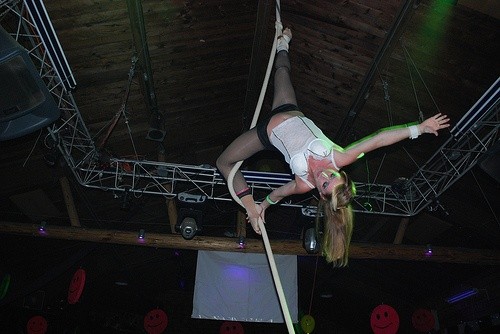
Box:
[300,220,323,255]
[24,0,78,92]
[175,209,205,240]
[450,77,500,141]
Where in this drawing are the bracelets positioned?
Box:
[409,125,418,139]
[266,194,278,205]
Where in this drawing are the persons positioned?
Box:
[216,28,450,268]
